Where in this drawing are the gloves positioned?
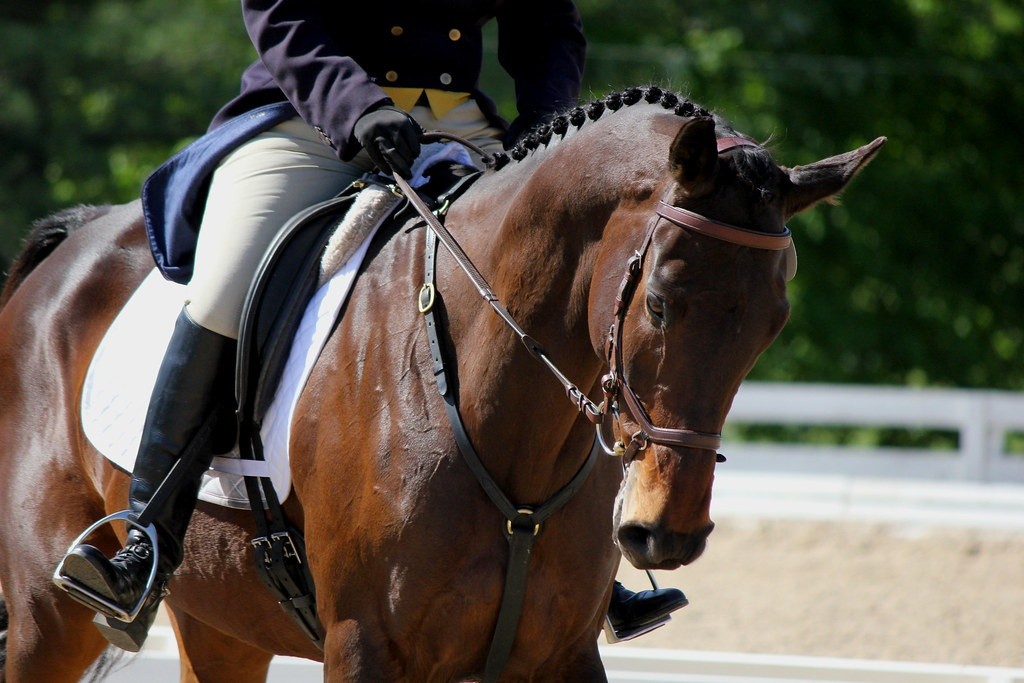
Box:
[352,105,422,181]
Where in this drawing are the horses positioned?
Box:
[1,80,889,683]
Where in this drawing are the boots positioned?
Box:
[607,582,687,638]
[64,299,245,651]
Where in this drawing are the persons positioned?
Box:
[62,0,689,652]
